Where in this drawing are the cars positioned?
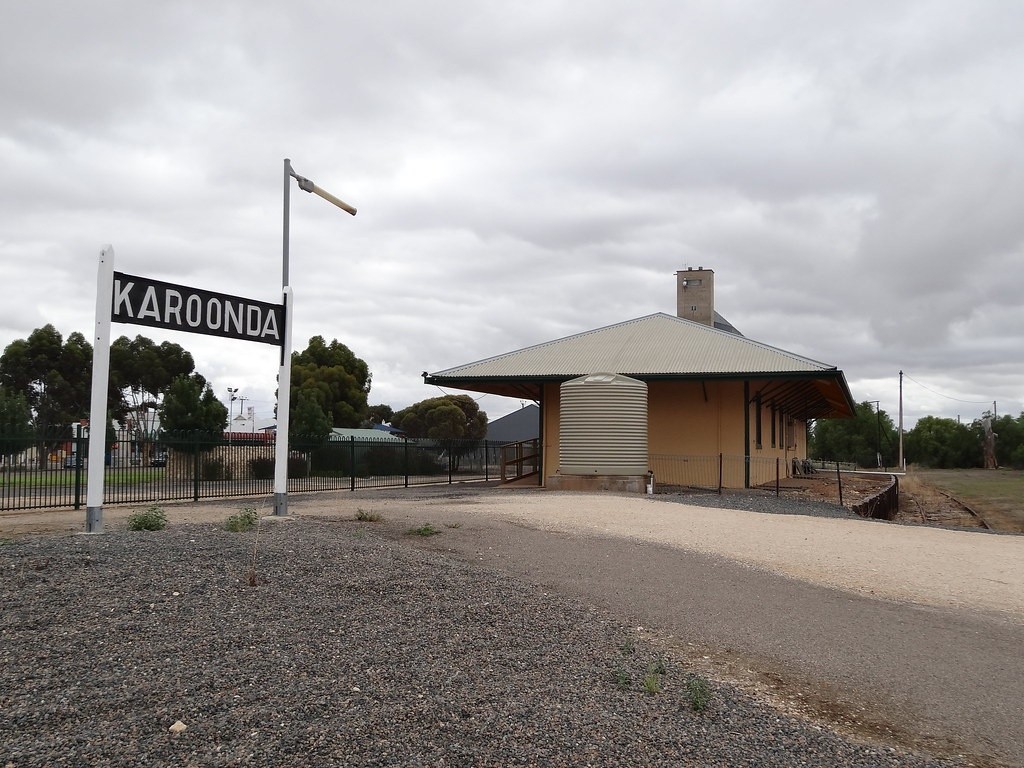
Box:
[61,451,84,469]
[130,456,142,465]
[151,456,168,467]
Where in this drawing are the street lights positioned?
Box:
[228,388,238,446]
[869,400,881,468]
